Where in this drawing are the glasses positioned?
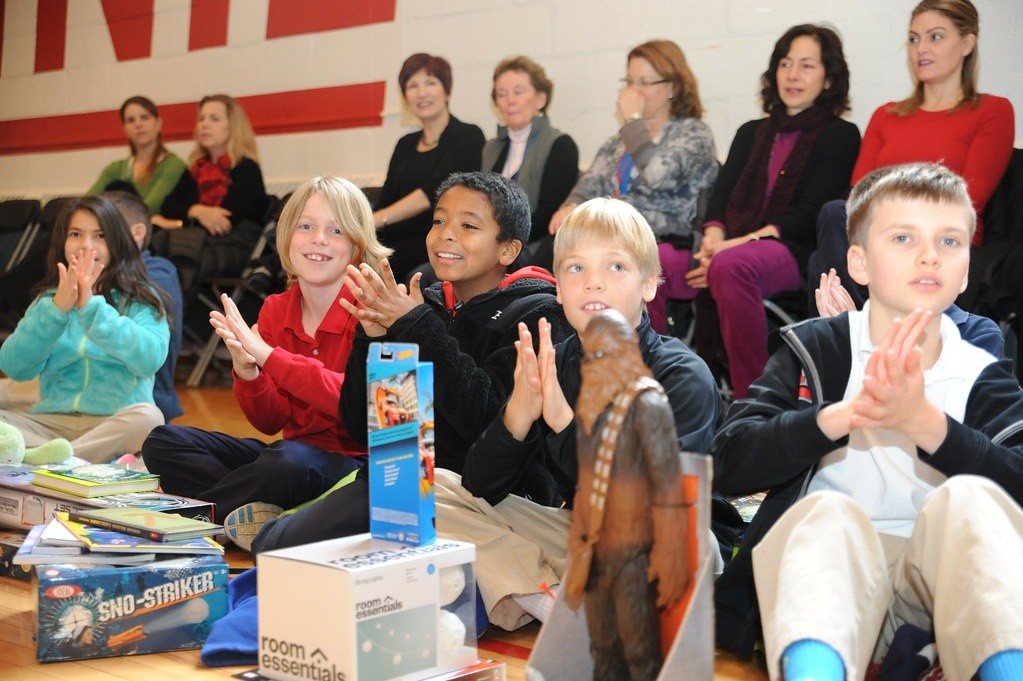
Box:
[619,76,670,89]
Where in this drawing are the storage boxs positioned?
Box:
[366,341,436,546]
[257,533,477,681]
[0,466,229,663]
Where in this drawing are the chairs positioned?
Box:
[0,192,293,389]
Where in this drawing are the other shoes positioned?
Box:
[223,501,284,551]
[110,453,138,465]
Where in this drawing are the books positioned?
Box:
[32,464,159,498]
[12,508,225,566]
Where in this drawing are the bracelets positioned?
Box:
[624,114,640,124]
[177,219,182,230]
[381,210,388,226]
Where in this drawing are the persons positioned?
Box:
[142,174,391,551]
[712,162,1022,681]
[686,25,863,398]
[810,0,1016,308]
[536,41,716,331]
[405,57,581,292]
[432,196,725,628]
[370,54,486,278]
[245,169,568,563]
[84,97,184,210]
[102,189,184,422]
[151,94,267,303]
[0,196,172,467]
[565,311,687,681]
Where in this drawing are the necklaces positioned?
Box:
[423,135,438,145]
